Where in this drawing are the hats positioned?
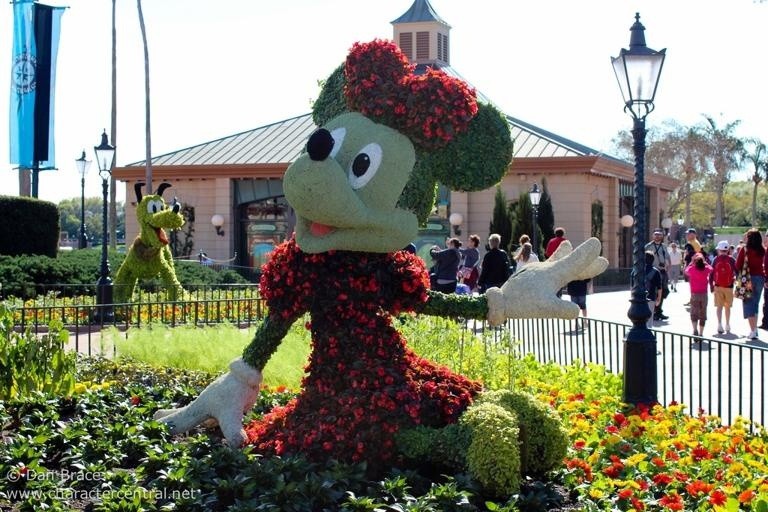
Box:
[653,228,663,235]
[685,229,696,233]
[715,241,730,250]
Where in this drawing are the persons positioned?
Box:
[407,228,768,345]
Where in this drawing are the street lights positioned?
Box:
[86,128,123,332]
[606,5,667,415]
[72,144,97,253]
[526,179,541,255]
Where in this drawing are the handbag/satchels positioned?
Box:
[733,263,753,299]
[457,265,478,282]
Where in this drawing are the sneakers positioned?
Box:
[693,322,768,341]
[654,312,669,320]
